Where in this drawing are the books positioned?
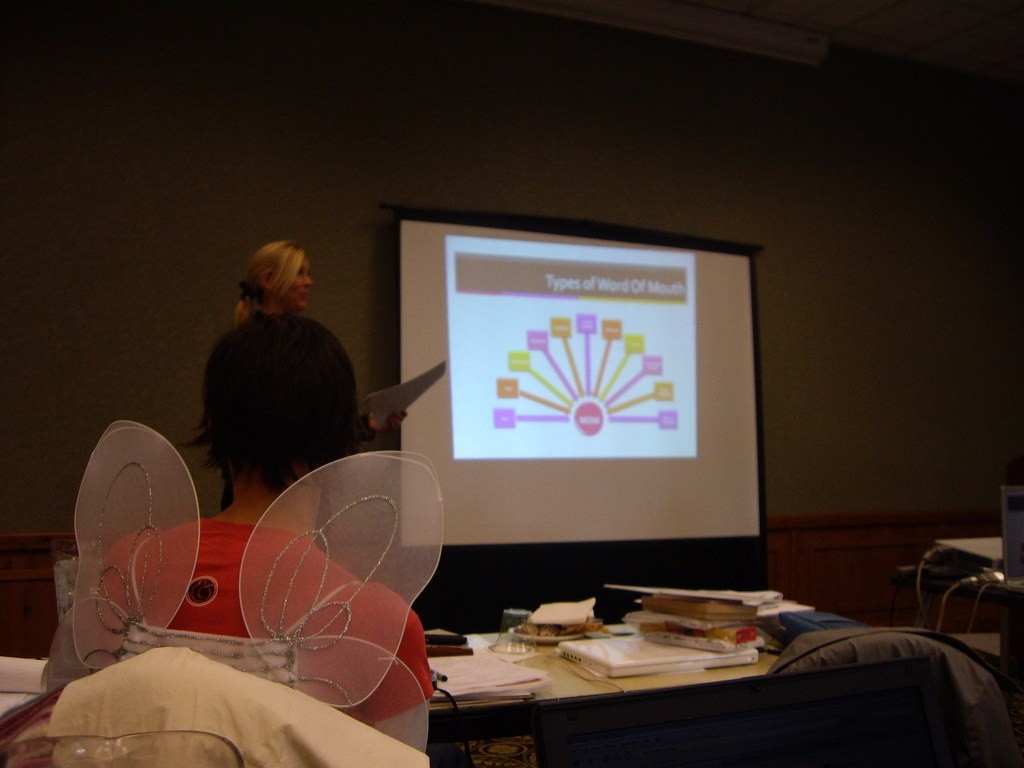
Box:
[421,627,473,657]
[641,595,758,621]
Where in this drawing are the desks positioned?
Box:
[424,622,783,768]
[894,571,1024,690]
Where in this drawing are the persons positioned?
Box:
[88,311,433,753]
[219,241,411,514]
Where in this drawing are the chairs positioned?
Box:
[535,654,971,768]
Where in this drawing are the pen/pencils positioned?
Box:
[430,668,448,682]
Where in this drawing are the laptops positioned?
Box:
[1001,485,1024,593]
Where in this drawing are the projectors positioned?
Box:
[932,536,1004,576]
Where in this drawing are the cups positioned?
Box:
[490,609,540,657]
